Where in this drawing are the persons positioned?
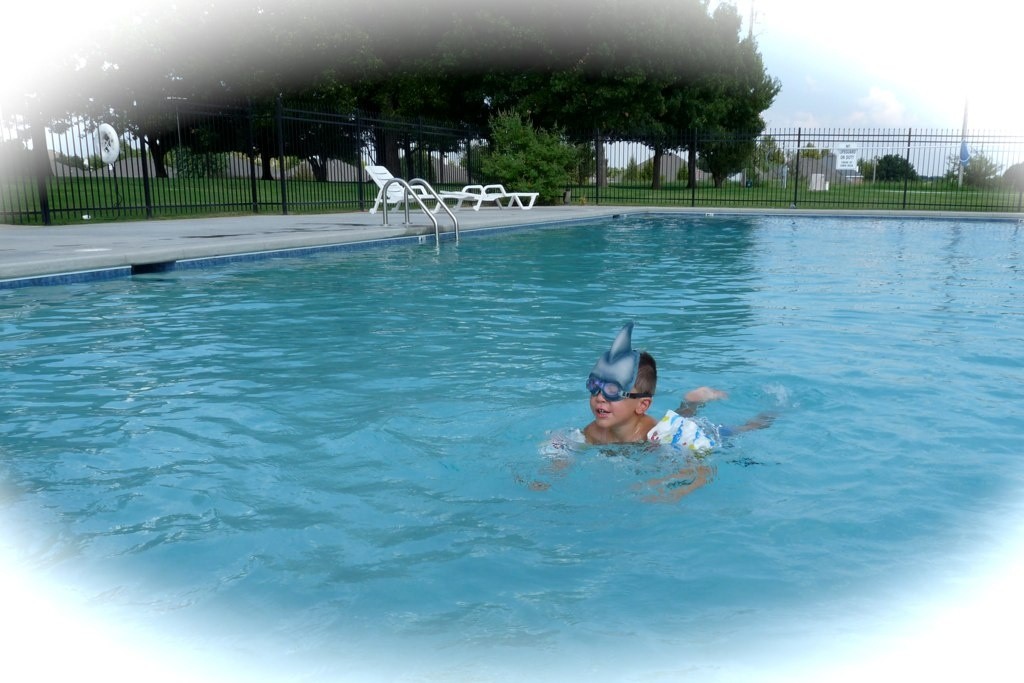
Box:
[583,321,714,450]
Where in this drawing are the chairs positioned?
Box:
[365,165,540,214]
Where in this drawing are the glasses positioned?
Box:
[586,377,631,402]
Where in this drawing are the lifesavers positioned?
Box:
[94,123,120,164]
